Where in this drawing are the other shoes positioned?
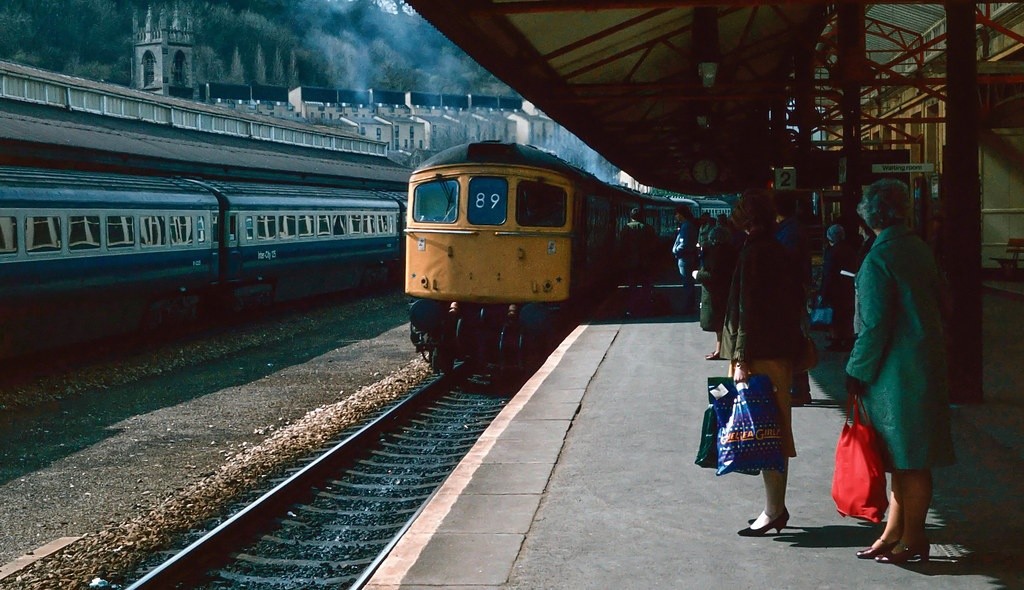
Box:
[705,352,720,359]
[791,392,811,407]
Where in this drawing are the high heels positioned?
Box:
[749,507,789,528]
[738,511,786,537]
[876,541,930,563]
[856,538,900,558]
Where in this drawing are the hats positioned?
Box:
[827,224,846,242]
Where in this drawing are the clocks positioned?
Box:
[692,158,719,185]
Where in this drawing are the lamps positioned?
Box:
[700,60,717,88]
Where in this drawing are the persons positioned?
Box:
[720,193,806,538]
[845,179,952,563]
[673,167,982,407]
[619,208,659,317]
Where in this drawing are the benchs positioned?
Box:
[988,238,1024,280]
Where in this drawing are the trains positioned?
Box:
[404,140,731,375]
[0,165,420,359]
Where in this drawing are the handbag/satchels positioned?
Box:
[810,296,833,324]
[791,337,817,374]
[694,376,786,476]
[831,393,889,523]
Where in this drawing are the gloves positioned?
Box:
[846,374,865,397]
